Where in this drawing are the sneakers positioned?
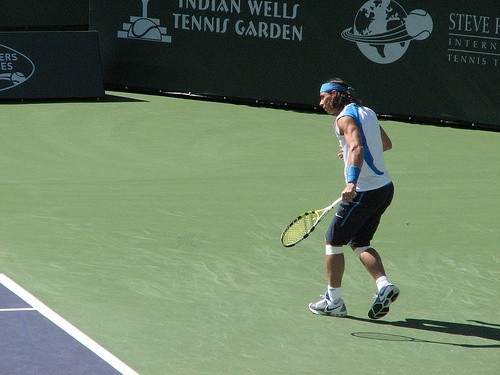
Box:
[368,283,400,319]
[309,299,347,317]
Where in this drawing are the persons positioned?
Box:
[309,78,400,319]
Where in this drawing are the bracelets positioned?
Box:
[347,166,360,182]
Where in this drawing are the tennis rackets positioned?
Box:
[280,191,358,248]
